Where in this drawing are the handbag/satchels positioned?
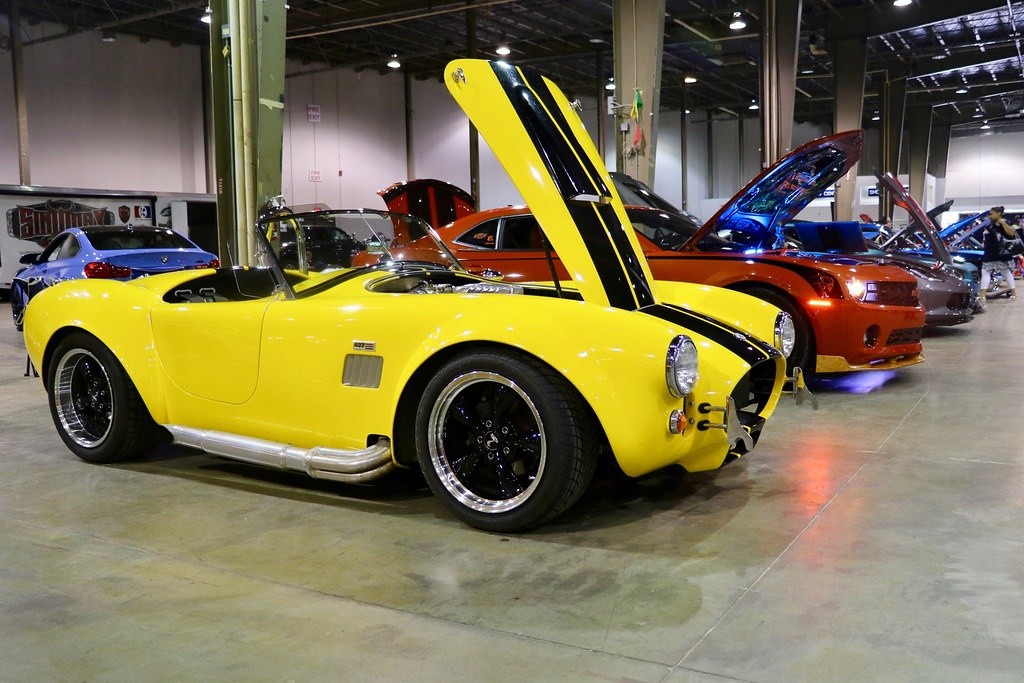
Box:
[996,224,1024,256]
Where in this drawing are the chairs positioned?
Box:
[219,264,278,301]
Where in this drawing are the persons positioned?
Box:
[872,216,892,244]
[974,205,1018,303]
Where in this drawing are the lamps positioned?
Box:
[872,107,881,121]
[893,0,912,6]
[684,67,697,84]
[495,30,511,55]
[605,76,616,89]
[980,117,991,129]
[387,50,400,69]
[748,99,760,110]
[956,84,969,94]
[972,106,984,118]
[728,10,746,29]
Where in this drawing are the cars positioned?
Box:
[23,53,795,535]
[782,200,989,307]
[9,224,221,331]
[793,169,972,329]
[350,128,925,393]
[277,219,367,275]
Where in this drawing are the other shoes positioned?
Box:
[1009,295,1017,300]
